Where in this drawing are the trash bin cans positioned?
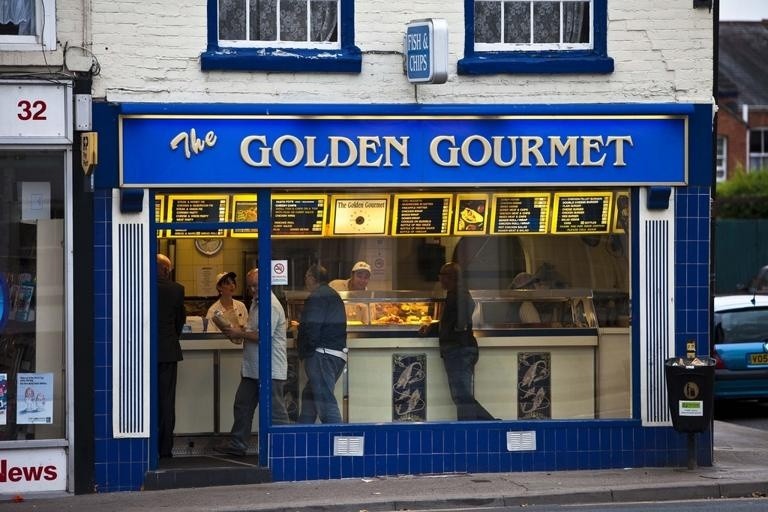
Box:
[664,356,717,435]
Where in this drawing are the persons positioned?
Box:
[419,262,504,420]
[329,261,373,291]
[505,271,548,321]
[297,264,347,425]
[155,253,186,461]
[213,268,292,459]
[205,271,248,333]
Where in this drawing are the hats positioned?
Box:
[512,272,542,288]
[351,260,373,275]
[214,271,237,287]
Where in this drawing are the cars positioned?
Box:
[711,292,768,421]
[734,263,768,294]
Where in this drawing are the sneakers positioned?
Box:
[212,440,247,459]
